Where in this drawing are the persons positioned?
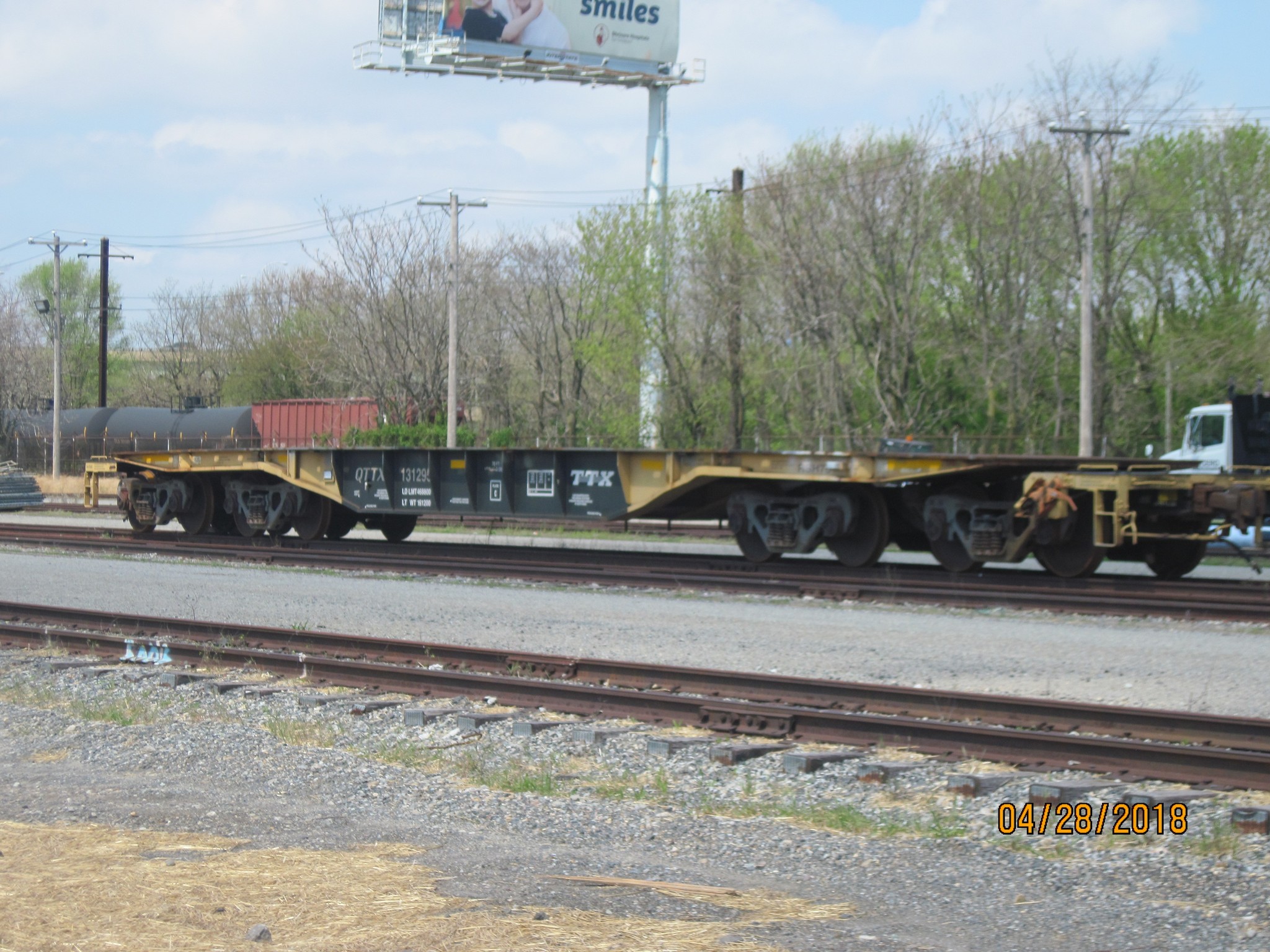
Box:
[461,0,570,48]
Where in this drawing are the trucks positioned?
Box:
[1143,385,1270,549]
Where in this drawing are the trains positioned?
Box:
[1,364,466,497]
